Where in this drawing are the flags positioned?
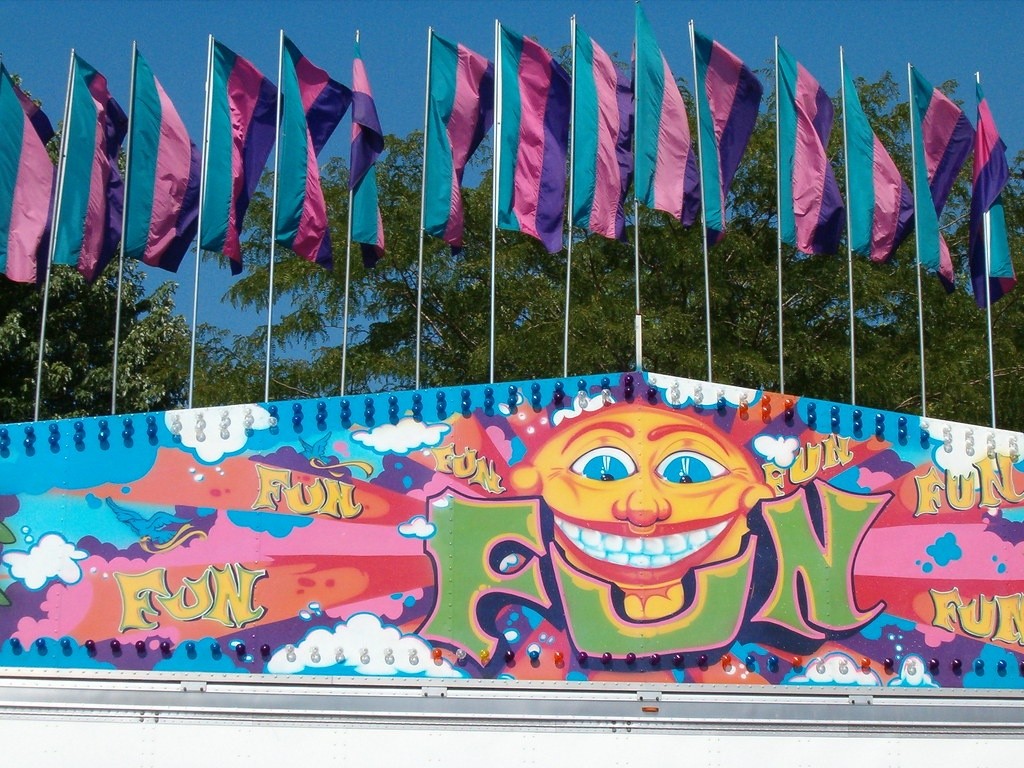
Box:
[1,1,1017,307]
